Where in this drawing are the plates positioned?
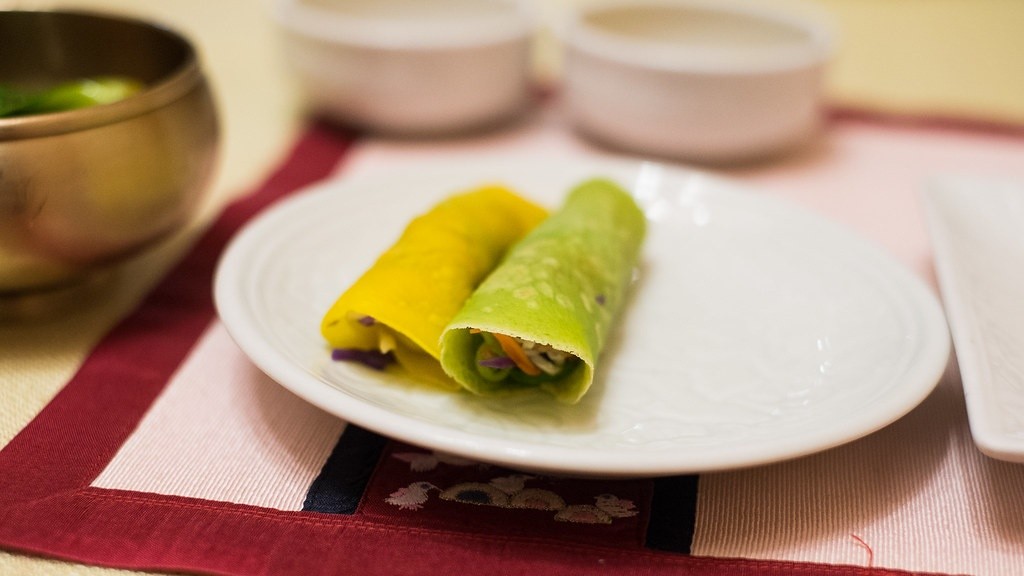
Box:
[926,174,1024,465]
[212,154,953,477]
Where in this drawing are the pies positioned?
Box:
[437,177,649,404]
[321,181,553,396]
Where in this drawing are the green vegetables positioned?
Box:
[0,70,146,121]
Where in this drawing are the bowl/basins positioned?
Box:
[551,0,832,168]
[0,11,222,324]
[278,0,537,135]
